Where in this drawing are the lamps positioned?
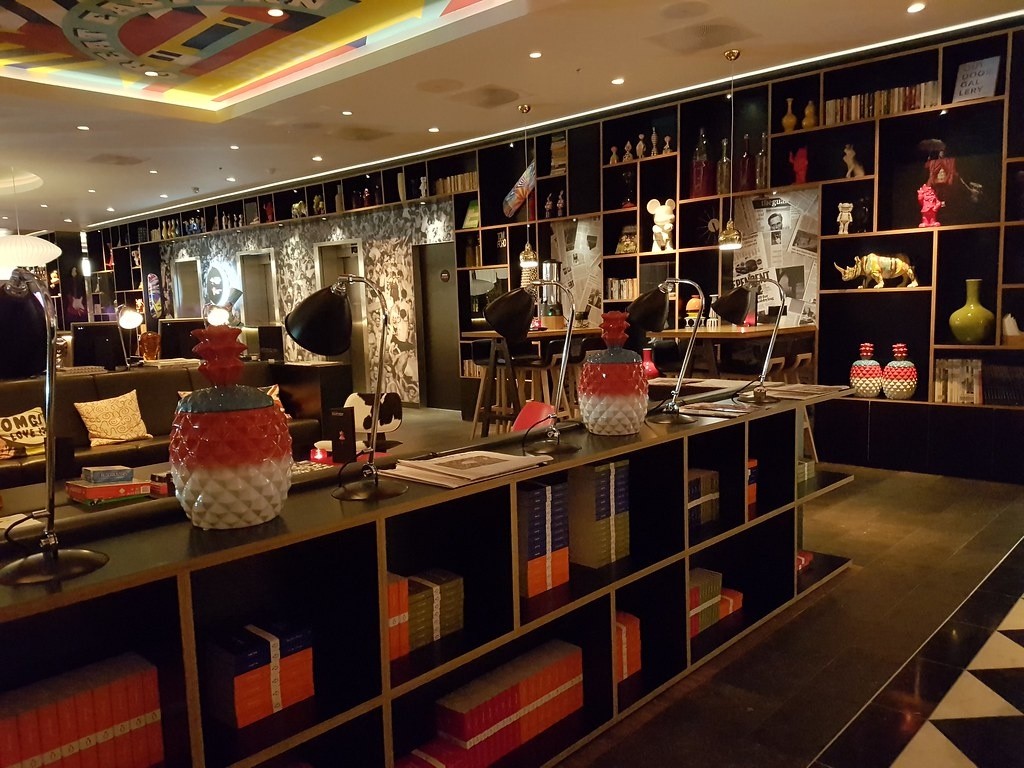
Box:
[711,278,786,402]
[0,268,111,586]
[0,166,61,267]
[484,280,584,456]
[114,304,143,369]
[627,277,704,425]
[282,274,406,502]
[516,104,538,269]
[718,49,742,250]
[202,300,229,328]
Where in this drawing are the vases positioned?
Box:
[617,172,636,208]
[948,279,994,345]
[781,99,796,132]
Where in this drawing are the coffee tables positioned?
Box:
[0,460,172,519]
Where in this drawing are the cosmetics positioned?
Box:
[463,358,482,377]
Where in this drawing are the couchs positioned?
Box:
[0,362,320,491]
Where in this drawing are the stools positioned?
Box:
[508,339,544,433]
[505,339,574,433]
[720,341,788,383]
[552,335,600,420]
[469,337,518,439]
[782,337,820,464]
[652,338,710,380]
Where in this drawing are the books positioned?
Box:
[605,277,638,300]
[0,652,163,768]
[688,467,720,530]
[394,639,583,768]
[435,171,479,195]
[690,567,743,638]
[823,81,936,126]
[386,571,464,660]
[550,133,567,174]
[678,403,764,418]
[935,358,1024,405]
[200,611,315,729]
[797,457,816,483]
[748,458,757,505]
[737,383,850,400]
[516,457,630,599]
[796,549,813,571]
[616,611,642,683]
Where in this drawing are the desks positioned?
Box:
[462,325,602,438]
[646,323,815,379]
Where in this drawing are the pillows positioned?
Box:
[0,406,47,460]
[73,388,155,447]
[257,384,293,421]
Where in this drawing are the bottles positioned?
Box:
[781,99,796,131]
[802,101,817,128]
[949,278,994,344]
[685,295,701,317]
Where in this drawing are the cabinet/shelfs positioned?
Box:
[0,10,1024,768]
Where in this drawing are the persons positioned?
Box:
[917,149,956,228]
[768,213,782,231]
[419,177,427,198]
[789,147,808,184]
[837,203,853,234]
[844,144,856,178]
